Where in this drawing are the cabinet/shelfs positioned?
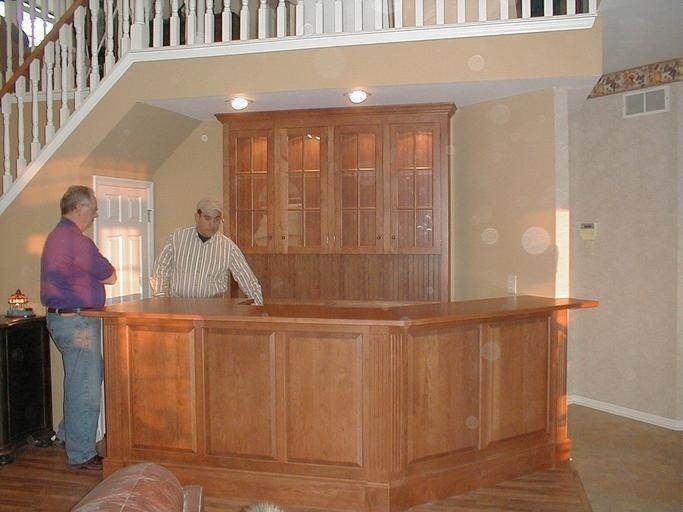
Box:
[1,314,56,467]
[217,102,458,256]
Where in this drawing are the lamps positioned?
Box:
[224,96,253,111]
[343,89,372,104]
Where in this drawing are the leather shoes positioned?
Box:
[51,436,66,451]
[64,454,105,471]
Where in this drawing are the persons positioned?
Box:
[149,198,263,307]
[39,184,118,471]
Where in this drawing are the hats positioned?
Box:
[196,197,223,214]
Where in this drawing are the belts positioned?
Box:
[47,307,95,314]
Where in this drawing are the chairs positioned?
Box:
[69,461,205,511]
